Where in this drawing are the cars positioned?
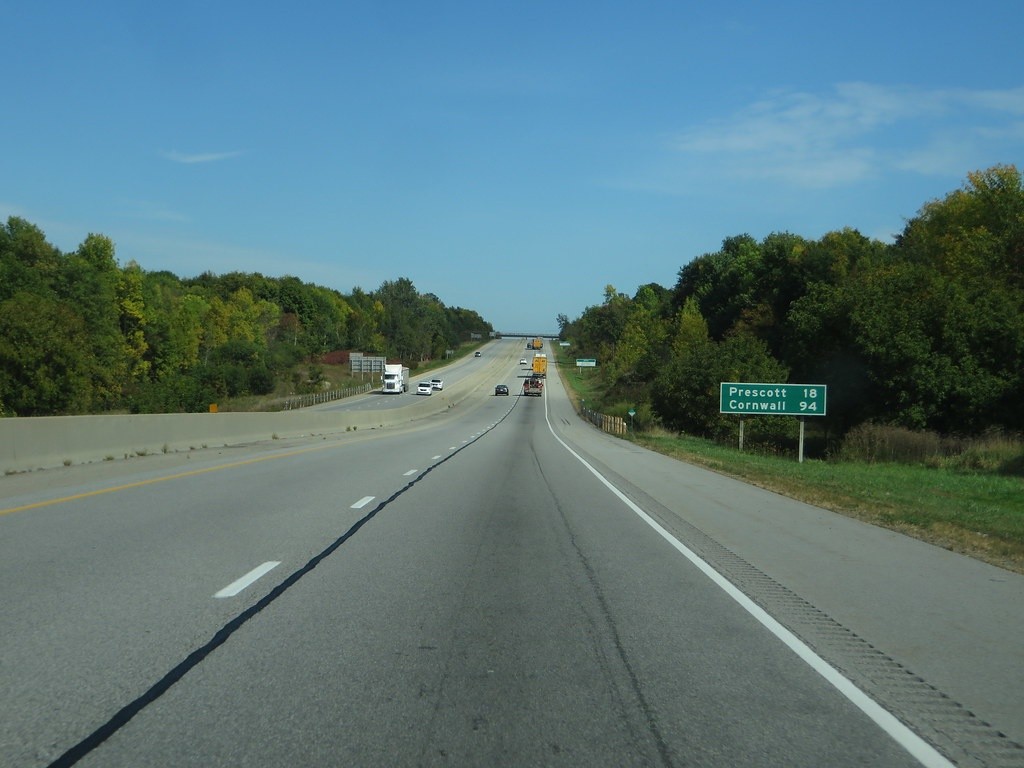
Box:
[475,352,481,357]
[495,385,508,396]
[430,379,443,390]
[417,382,432,395]
[520,358,527,365]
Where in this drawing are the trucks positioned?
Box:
[533,339,541,350]
[524,378,543,396]
[381,364,409,394]
[532,354,547,379]
